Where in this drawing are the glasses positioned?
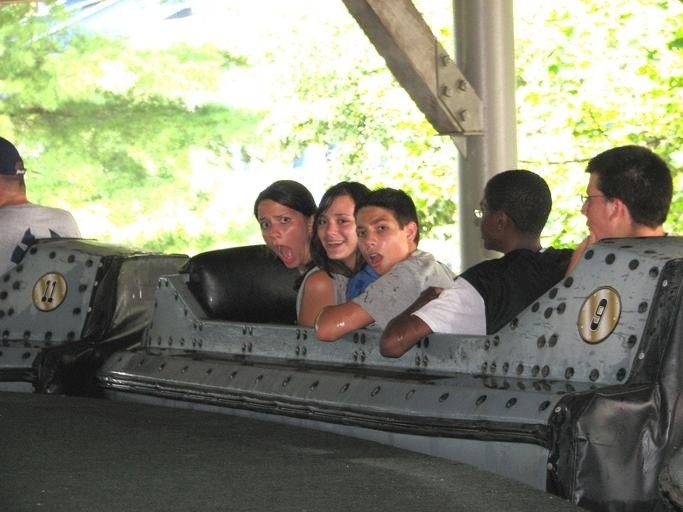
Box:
[579,193,605,204]
[473,207,495,220]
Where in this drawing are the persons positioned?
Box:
[0,136,77,282]
[563,143,670,276]
[297,181,371,328]
[253,179,318,327]
[313,185,456,344]
[378,168,576,360]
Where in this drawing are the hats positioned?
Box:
[0,135,26,177]
